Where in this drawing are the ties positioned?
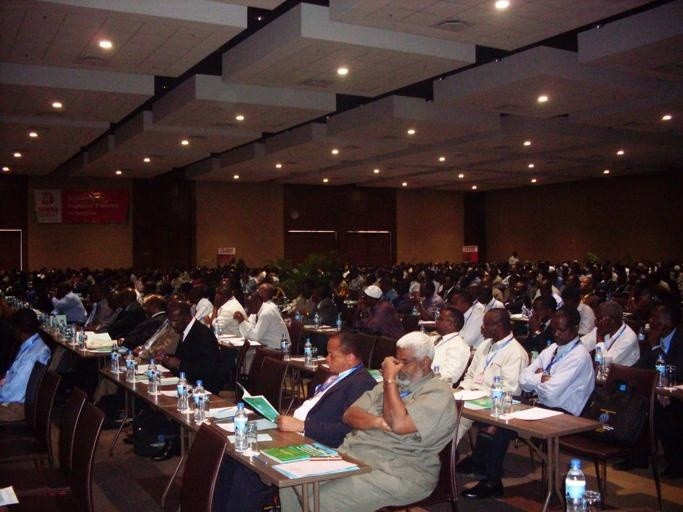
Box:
[433,337,442,346]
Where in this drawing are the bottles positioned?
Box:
[563,457,587,512]
[655,352,668,389]
[110,342,260,459]
[593,347,605,384]
[489,372,504,423]
[279,310,344,366]
[38,311,88,351]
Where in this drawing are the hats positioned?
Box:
[364,285,382,299]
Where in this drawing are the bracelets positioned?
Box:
[384,380,399,387]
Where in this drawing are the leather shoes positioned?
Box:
[152,443,181,461]
[612,459,649,470]
[662,466,682,480]
[462,480,504,499]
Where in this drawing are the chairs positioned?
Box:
[1,293,683,512]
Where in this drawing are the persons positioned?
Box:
[455,306,596,500]
[578,302,641,369]
[211,332,377,509]
[137,300,217,461]
[0,252,683,409]
[273,331,458,510]
[428,307,471,385]
[455,308,529,448]
[2,309,51,423]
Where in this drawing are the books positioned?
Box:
[261,443,339,464]
[237,382,280,422]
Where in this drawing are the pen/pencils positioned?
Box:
[219,408,232,412]
[310,456,342,461]
